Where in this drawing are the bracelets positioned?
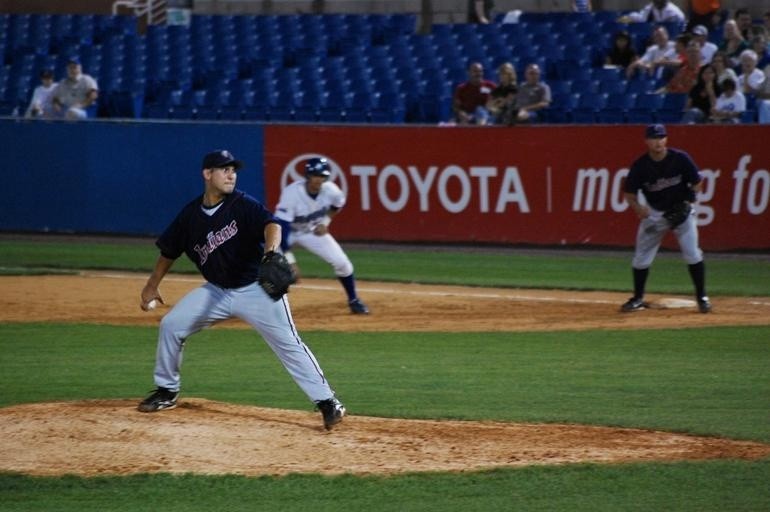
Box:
[319,213,336,228]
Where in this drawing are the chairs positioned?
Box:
[0,8,769,122]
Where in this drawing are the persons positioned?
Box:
[571,0,592,13]
[23,69,58,120]
[451,63,498,125]
[52,57,98,119]
[604,0,770,125]
[518,62,551,125]
[271,158,369,316]
[620,122,711,314]
[471,62,519,127]
[138,149,347,426]
[467,0,494,24]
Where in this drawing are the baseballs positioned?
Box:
[147,300,156,310]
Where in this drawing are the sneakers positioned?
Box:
[350,299,368,314]
[139,387,179,412]
[622,298,645,311]
[699,296,711,313]
[318,396,346,431]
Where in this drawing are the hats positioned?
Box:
[203,150,242,171]
[647,125,666,137]
[693,25,708,37]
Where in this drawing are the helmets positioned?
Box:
[306,158,331,177]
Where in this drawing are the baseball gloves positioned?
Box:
[662,200,691,229]
[257,250,298,300]
